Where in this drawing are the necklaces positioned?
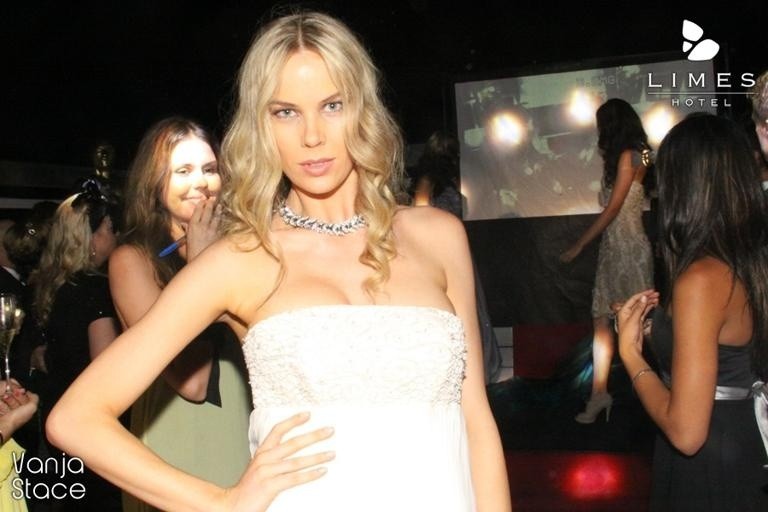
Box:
[271,185,367,236]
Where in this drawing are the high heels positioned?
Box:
[575,395,614,424]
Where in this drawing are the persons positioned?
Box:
[46,12,511,512]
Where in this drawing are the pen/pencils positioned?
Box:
[158,235,187,258]
